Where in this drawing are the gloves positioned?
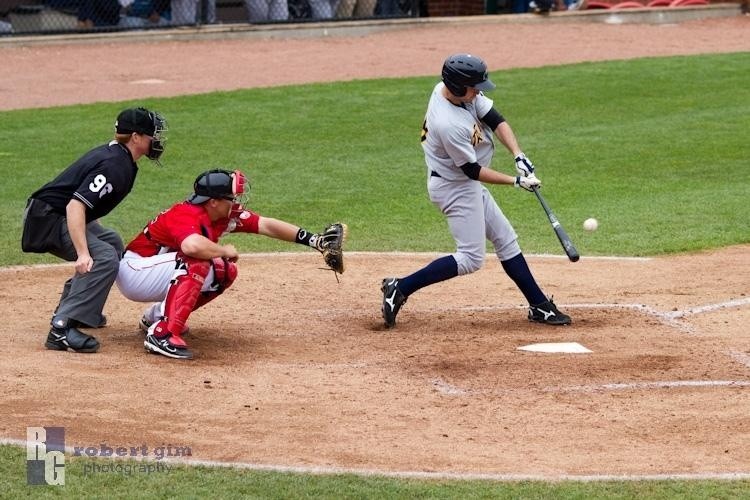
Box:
[514,153,541,192]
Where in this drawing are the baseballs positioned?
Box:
[585,218,598,232]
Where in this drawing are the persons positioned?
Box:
[22,107,169,351]
[381,51,581,327]
[116,168,346,359]
[0,1,554,32]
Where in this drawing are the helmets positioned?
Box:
[115,107,169,160]
[188,169,251,221]
[441,53,496,97]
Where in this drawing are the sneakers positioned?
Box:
[45,327,100,352]
[527,294,572,325]
[50,307,106,328]
[381,277,408,327]
[143,332,193,360]
[139,314,189,336]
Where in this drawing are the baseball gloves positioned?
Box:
[316,222,346,274]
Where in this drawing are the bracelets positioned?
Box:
[296,229,312,245]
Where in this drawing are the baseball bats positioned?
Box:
[532,184,580,262]
